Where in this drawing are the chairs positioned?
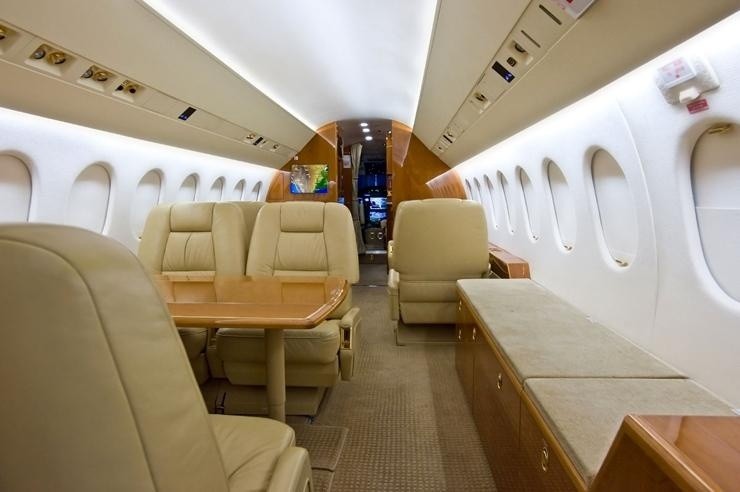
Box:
[0,200,362,492]
[387,197,502,346]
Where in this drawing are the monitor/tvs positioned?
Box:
[369,212,387,225]
[370,197,388,209]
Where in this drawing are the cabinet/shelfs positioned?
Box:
[519,382,587,491]
[472,325,522,492]
[450,290,475,405]
